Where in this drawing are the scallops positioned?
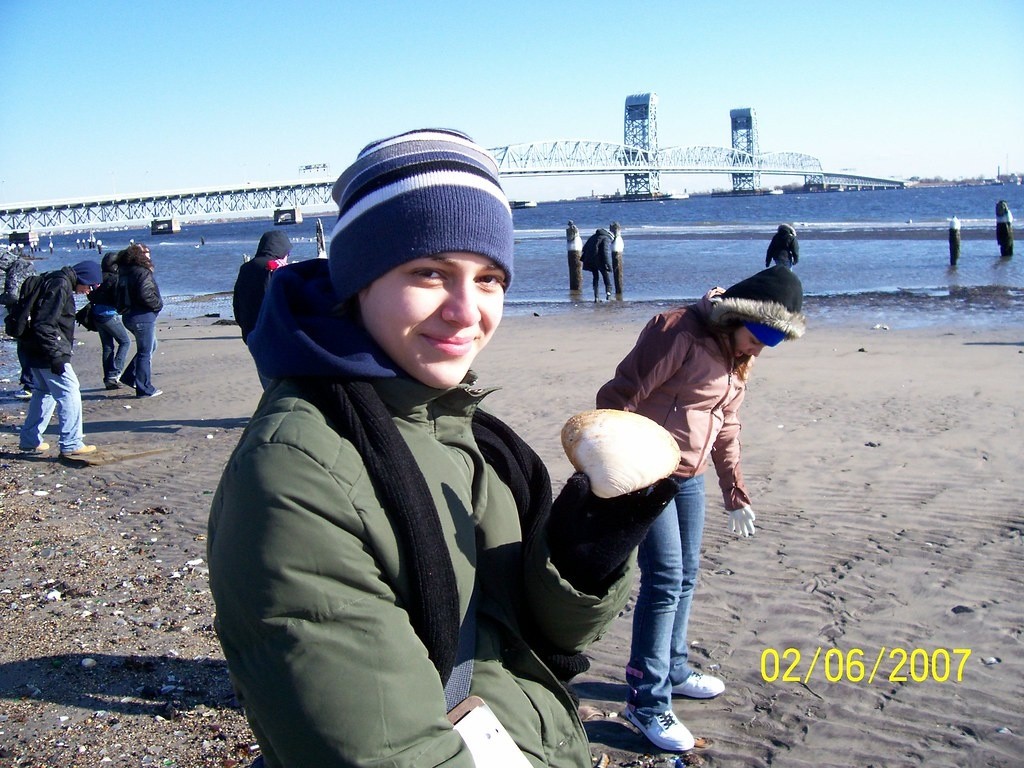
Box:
[560,409,680,498]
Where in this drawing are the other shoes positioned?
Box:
[60,445,95,457]
[102,375,123,389]
[137,389,162,399]
[14,386,32,397]
[19,442,50,451]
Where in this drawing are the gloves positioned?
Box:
[607,264,612,272]
[541,472,682,590]
[50,356,66,377]
[726,503,756,537]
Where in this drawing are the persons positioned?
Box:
[597,265,806,751]
[579,227,615,302]
[77,237,102,255]
[994,201,1014,257]
[608,222,624,294]
[87,243,164,398]
[11,241,53,255]
[206,128,681,768]
[949,217,961,265]
[765,223,799,272]
[0,250,104,457]
[232,231,294,344]
[565,221,583,291]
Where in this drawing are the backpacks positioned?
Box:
[3,271,71,343]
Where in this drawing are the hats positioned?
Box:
[328,128,514,301]
[607,230,615,240]
[747,320,787,347]
[133,243,151,254]
[92,283,102,292]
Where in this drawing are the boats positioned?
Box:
[990,183,1004,186]
[509,199,537,210]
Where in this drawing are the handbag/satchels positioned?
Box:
[75,302,97,332]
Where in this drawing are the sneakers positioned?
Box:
[625,702,695,750]
[670,673,725,698]
